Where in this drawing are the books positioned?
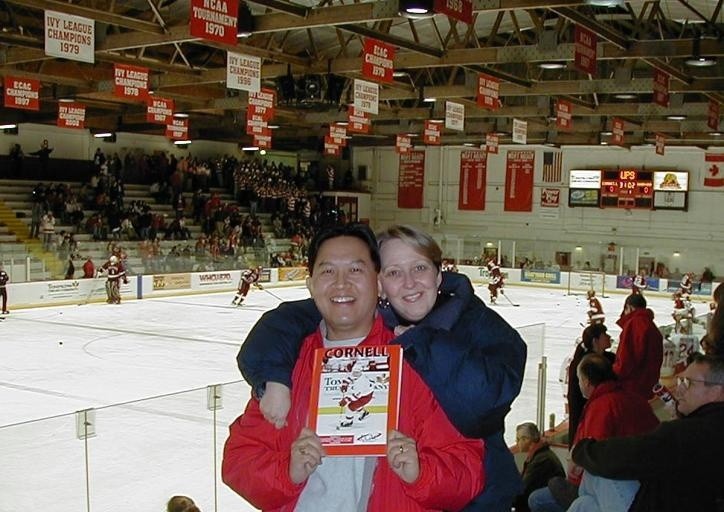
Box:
[307,343,406,456]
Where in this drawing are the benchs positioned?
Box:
[0,178,293,285]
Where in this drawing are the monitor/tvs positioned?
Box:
[653,170,689,192]
[569,169,602,190]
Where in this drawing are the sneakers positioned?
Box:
[3,311,9,314]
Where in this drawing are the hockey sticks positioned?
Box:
[579,317,589,328]
[78,276,99,306]
[501,290,520,307]
[336,389,345,430]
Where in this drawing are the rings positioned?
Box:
[299,448,305,455]
[399,444,404,454]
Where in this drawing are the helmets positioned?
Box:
[675,291,682,297]
[588,290,595,297]
[109,255,117,262]
[687,272,692,277]
[640,270,645,276]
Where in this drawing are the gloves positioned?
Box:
[124,280,128,284]
[100,269,103,273]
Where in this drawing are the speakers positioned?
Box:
[276,72,348,106]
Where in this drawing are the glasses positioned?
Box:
[676,376,722,389]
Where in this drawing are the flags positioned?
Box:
[542,151,563,182]
[703,151,724,187]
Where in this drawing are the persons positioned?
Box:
[338,362,374,427]
[441,258,503,305]
[234,221,529,512]
[231,264,264,307]
[96,255,129,305]
[220,217,487,511]
[11,137,350,281]
[0,261,10,314]
[512,253,723,511]
